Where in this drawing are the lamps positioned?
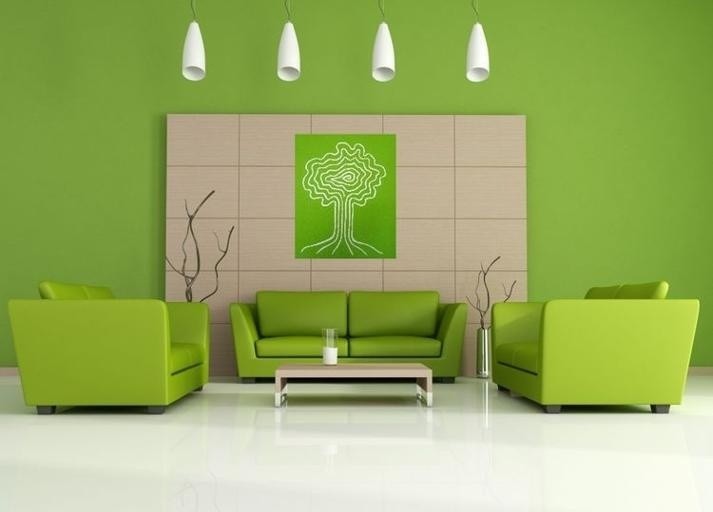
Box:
[181,0,206,81]
[371,0,395,82]
[466,0,490,83]
[277,0,301,82]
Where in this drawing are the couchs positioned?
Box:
[491,281,700,414]
[230,290,468,383]
[8,282,210,414]
